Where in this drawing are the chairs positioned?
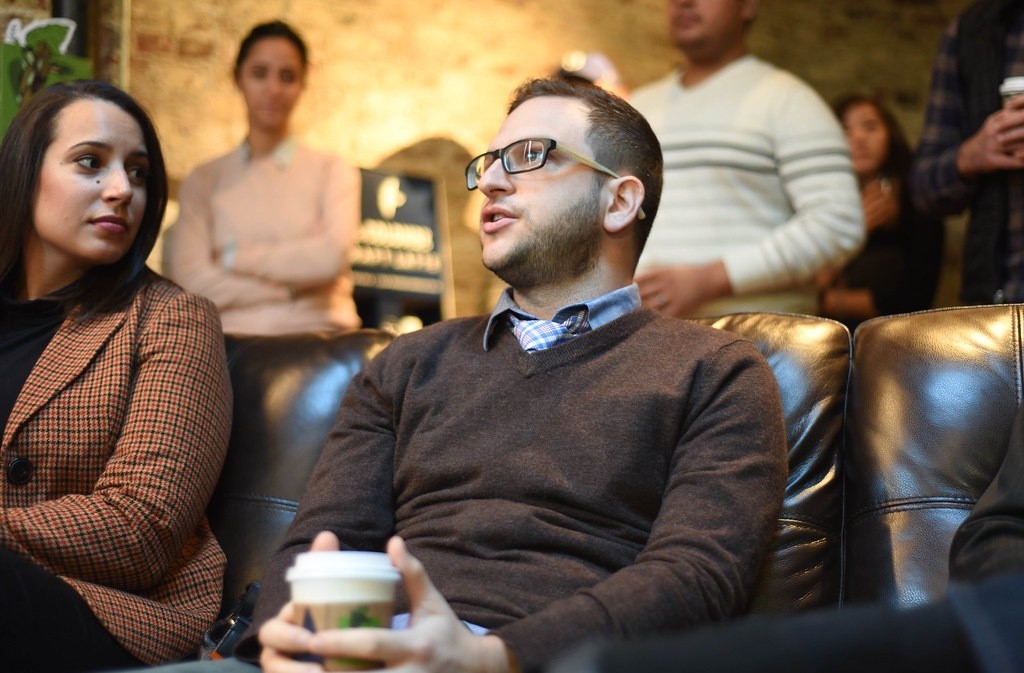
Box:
[200,330,394,673]
[840,301,1024,611]
[674,313,855,621]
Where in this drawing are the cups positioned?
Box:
[999,78,1024,110]
[283,552,402,672]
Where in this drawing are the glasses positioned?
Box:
[465,137,646,220]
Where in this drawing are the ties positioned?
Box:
[505,308,592,355]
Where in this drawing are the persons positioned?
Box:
[818,92,944,333]
[0,79,232,673]
[623,0,867,321]
[134,72,785,673]
[168,21,361,336]
[909,0,1024,306]
